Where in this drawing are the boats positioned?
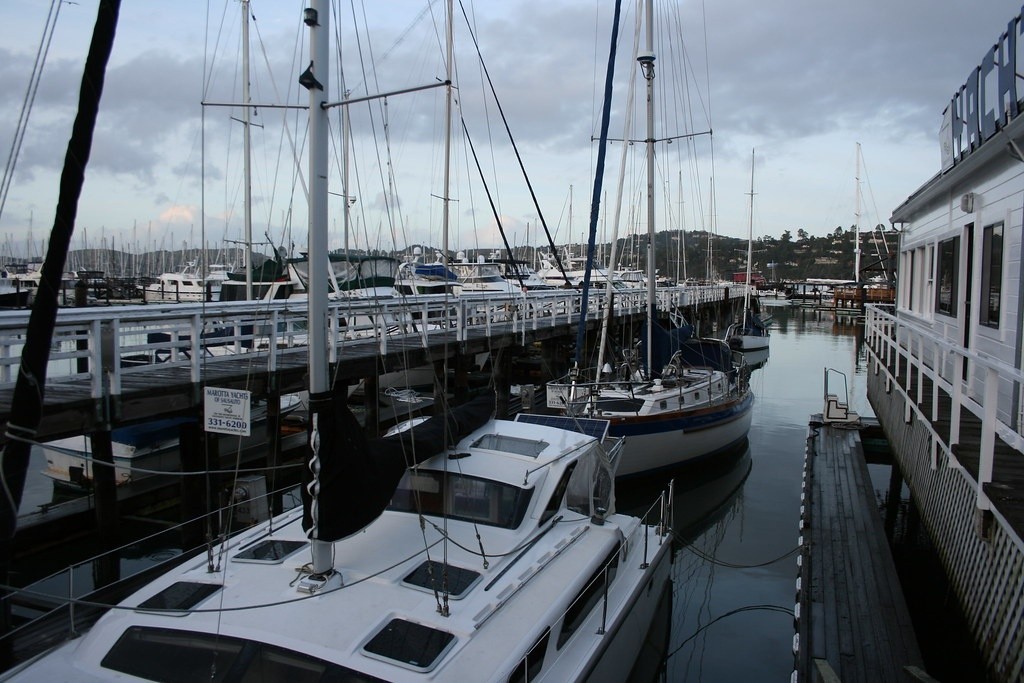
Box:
[43,389,303,489]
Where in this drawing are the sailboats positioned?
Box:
[2,0,684,682]
[495,0,755,488]
[75,141,898,372]
